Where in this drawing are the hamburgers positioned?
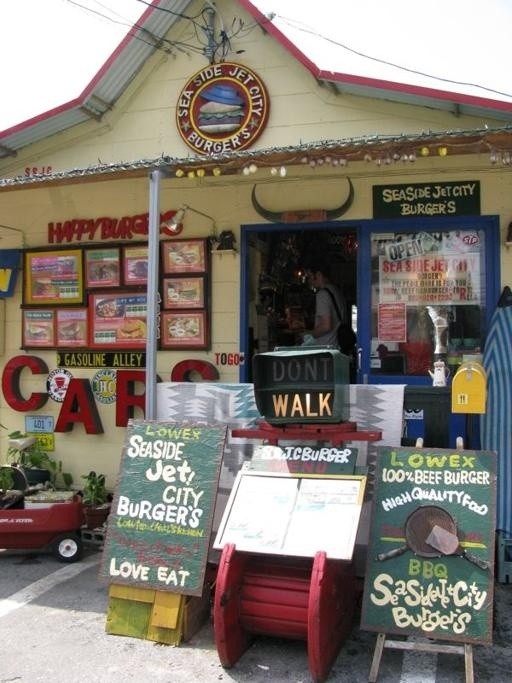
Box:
[198,102,245,132]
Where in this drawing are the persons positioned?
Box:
[293,258,346,346]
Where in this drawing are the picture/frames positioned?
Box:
[20,236,212,351]
[212,468,367,564]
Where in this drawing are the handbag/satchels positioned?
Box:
[336,319,358,355]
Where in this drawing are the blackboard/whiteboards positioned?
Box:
[372,180,480,216]
[359,447,498,647]
[249,445,358,477]
[97,418,227,598]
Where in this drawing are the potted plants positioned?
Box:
[0,430,74,509]
[80,471,111,529]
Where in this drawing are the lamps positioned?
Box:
[160,203,218,244]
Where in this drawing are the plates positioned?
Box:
[94,297,126,319]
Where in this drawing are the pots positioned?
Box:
[374,505,458,562]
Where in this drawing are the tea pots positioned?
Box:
[427,359,450,387]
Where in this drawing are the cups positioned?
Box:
[463,338,476,347]
[450,338,462,346]
[169,326,185,337]
